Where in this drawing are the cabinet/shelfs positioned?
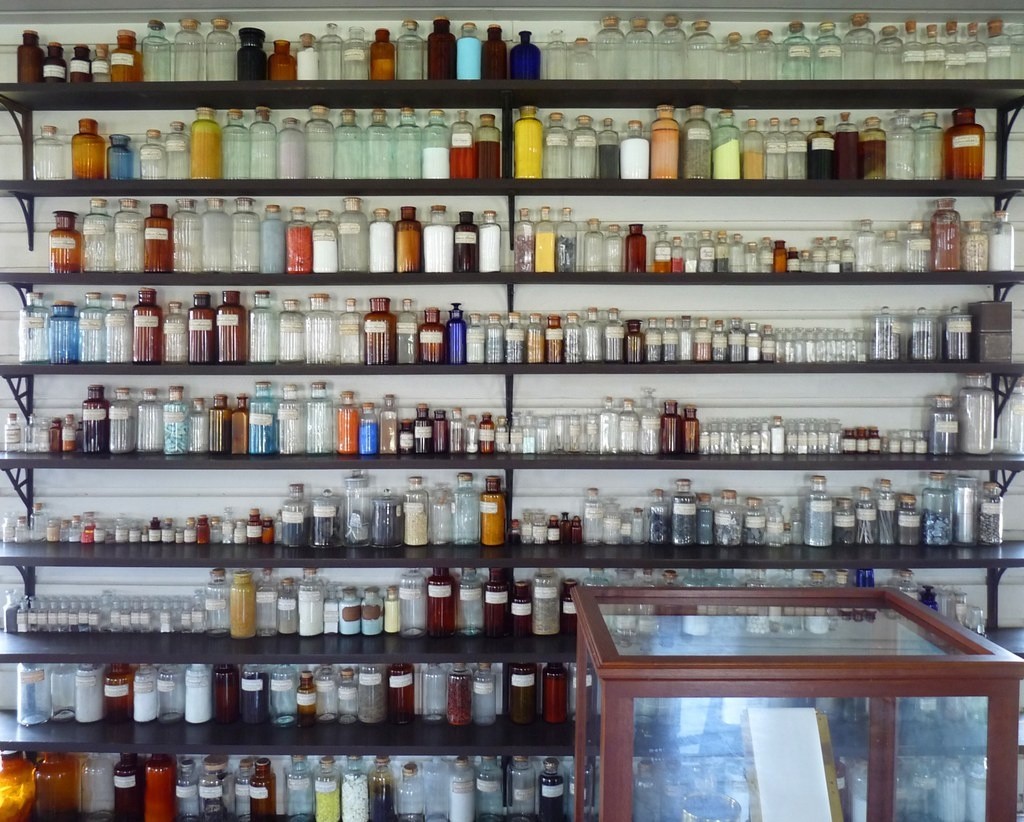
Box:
[0,0,1024,822]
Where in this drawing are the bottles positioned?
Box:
[0,9,1023,822]
